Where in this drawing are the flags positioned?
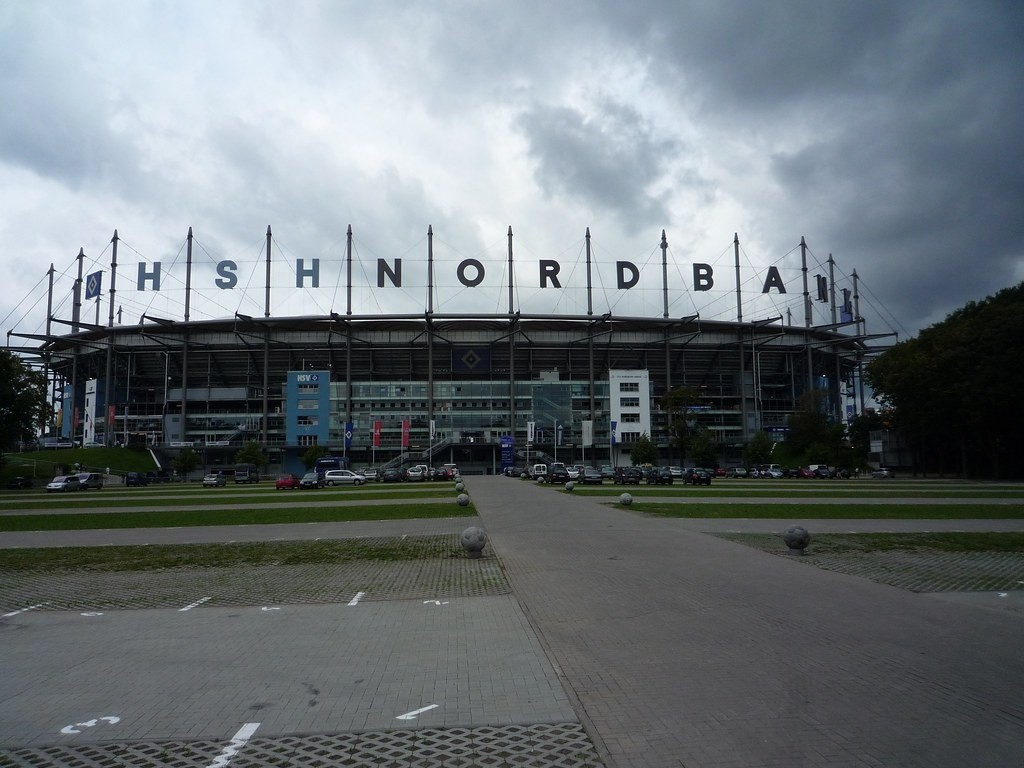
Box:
[611,421,617,445]
[403,420,409,446]
[345,423,353,447]
[58,409,62,427]
[528,422,535,441]
[374,422,381,446]
[75,408,79,427]
[109,406,115,424]
[430,420,436,440]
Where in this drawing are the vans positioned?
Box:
[46,473,103,492]
[126,472,147,486]
[234,463,259,483]
[532,464,546,480]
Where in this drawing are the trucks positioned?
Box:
[314,457,346,479]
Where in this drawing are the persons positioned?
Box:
[524,464,530,480]
[855,467,859,479]
[577,466,584,484]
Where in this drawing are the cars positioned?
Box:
[275,474,300,490]
[203,474,226,487]
[7,477,34,490]
[548,463,716,485]
[300,473,325,489]
[872,468,896,478]
[383,464,459,482]
[716,463,851,479]
[84,442,106,448]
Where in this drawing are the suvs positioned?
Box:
[325,467,381,486]
